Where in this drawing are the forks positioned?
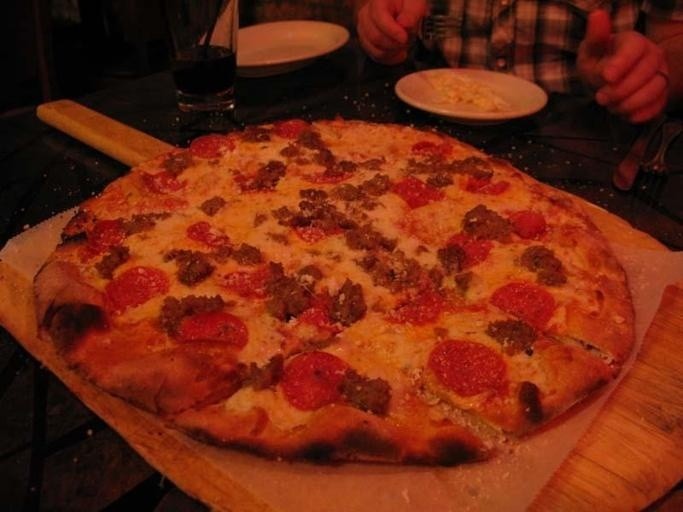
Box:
[643,122,683,174]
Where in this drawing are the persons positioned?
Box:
[356,0,683,124]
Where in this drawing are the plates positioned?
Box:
[236,20,349,77]
[394,67,547,125]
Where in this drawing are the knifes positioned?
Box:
[612,114,665,191]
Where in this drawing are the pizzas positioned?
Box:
[33,118,637,463]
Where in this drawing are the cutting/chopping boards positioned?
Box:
[1,100,683,511]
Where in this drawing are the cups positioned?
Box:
[166,1,238,113]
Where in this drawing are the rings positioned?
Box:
[656,70,670,83]
